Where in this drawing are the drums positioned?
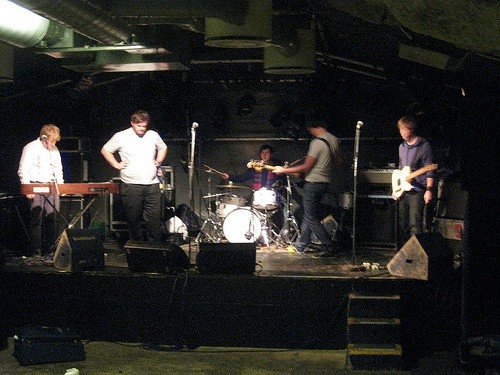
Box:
[253,189,276,210]
[222,206,266,243]
[217,196,248,217]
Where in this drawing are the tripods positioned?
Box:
[260,173,301,249]
[195,171,223,242]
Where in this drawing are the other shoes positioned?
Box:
[289,242,304,255]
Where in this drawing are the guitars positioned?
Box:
[392,163,438,201]
[246,158,304,180]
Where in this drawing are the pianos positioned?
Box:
[19,182,120,262]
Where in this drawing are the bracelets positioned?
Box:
[426,186,433,191]
[156,159,163,165]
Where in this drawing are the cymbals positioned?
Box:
[216,185,246,188]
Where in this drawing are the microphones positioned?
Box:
[191,122,199,131]
[356,121,363,129]
[246,233,251,240]
[42,134,48,141]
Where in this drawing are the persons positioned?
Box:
[17,124,65,256]
[222,144,301,238]
[101,110,167,242]
[397,116,434,250]
[272,116,344,260]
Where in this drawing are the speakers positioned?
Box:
[342,194,402,251]
[387,232,456,280]
[124,240,189,275]
[196,244,257,274]
[51,200,105,272]
